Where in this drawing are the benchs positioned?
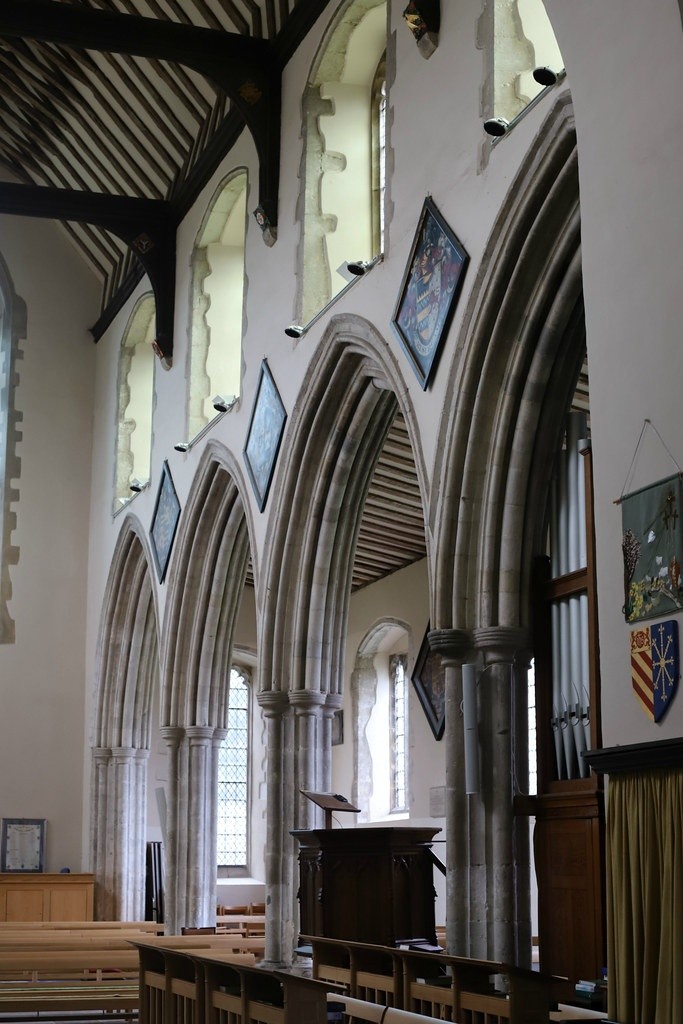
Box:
[0,915,266,1023]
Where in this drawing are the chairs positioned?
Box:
[215,901,265,958]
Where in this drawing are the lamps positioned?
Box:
[213,400,234,412]
[284,325,304,339]
[532,65,557,86]
[129,483,142,494]
[174,442,189,452]
[483,116,508,137]
[347,260,366,275]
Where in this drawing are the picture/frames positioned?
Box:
[410,617,445,741]
[0,818,47,873]
